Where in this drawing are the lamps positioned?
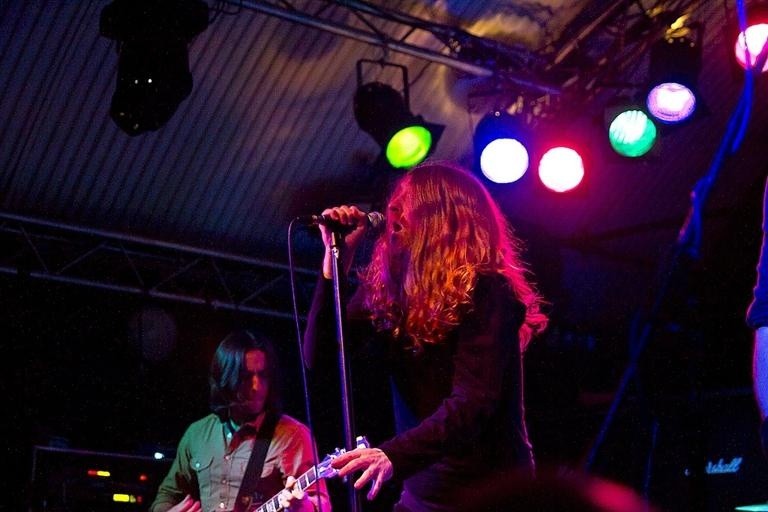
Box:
[469,89,528,185]
[537,139,593,201]
[732,11,768,75]
[603,104,658,159]
[644,27,698,126]
[352,58,446,173]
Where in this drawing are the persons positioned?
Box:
[303,161,536,512]
[149,329,332,512]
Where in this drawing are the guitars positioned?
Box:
[250,431,369,511]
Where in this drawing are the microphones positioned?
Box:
[296,211,383,232]
